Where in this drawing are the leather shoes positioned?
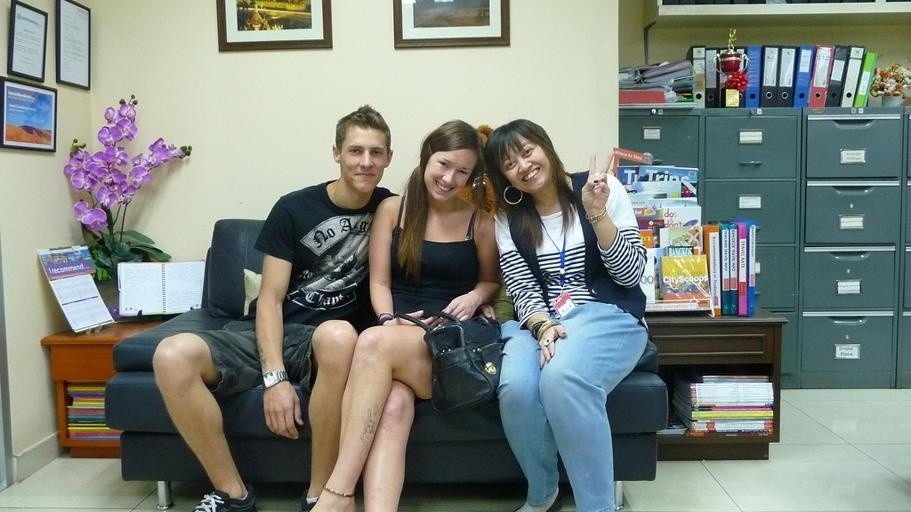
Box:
[514,491,561,512]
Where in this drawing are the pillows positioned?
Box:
[242,268,262,317]
[492,274,515,324]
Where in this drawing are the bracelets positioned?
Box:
[584,207,608,225]
[376,311,392,320]
[537,319,562,342]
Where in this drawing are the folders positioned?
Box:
[685,44,876,107]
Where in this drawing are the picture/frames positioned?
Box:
[0,0,91,152]
[393,0,509,48]
[216,0,333,50]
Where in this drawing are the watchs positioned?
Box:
[263,369,289,388]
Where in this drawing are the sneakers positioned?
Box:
[193,484,257,512]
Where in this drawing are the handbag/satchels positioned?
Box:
[424,316,504,413]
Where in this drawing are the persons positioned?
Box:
[154,105,494,511]
[311,119,501,511]
[483,118,648,511]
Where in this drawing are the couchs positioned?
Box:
[105,220,669,510]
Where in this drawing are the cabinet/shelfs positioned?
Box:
[620,107,910,388]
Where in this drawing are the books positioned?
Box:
[608,146,757,317]
[65,383,124,441]
[655,372,776,439]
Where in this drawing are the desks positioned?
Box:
[41,322,163,457]
[639,305,788,460]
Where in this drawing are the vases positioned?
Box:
[95,282,119,307]
[882,95,902,107]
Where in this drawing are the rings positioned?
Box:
[544,338,555,347]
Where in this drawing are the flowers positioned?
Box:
[869,64,911,99]
[63,93,192,282]
